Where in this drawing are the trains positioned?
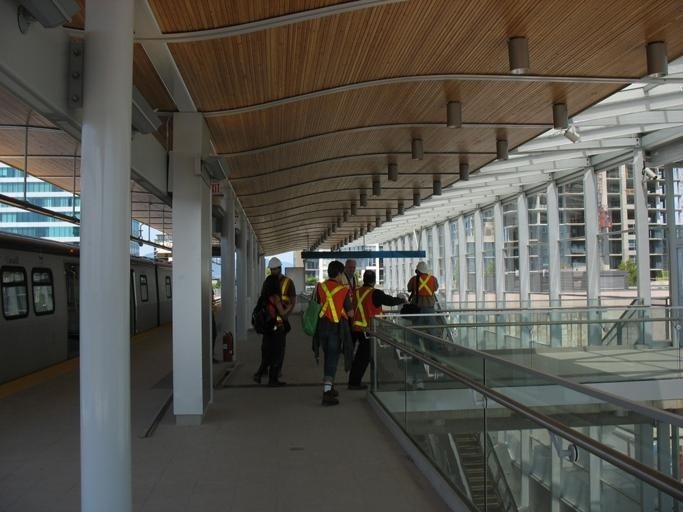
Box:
[0,228,173,392]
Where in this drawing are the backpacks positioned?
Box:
[250,295,275,333]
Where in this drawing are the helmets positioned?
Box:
[417,261,428,274]
[268,258,282,268]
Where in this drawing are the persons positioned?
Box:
[254,259,296,377]
[347,269,405,390]
[341,260,360,360]
[302,261,355,406]
[251,278,288,386]
[405,260,440,357]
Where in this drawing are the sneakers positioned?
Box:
[253,369,287,385]
[320,385,340,406]
[348,383,369,390]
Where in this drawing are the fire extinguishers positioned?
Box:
[222,330,233,362]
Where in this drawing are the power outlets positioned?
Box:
[564,130,578,141]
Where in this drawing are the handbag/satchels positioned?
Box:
[400,304,420,321]
[302,301,322,336]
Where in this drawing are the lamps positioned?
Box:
[496,140,507,161]
[648,41,667,78]
[553,103,568,129]
[412,139,422,160]
[447,101,461,128]
[508,37,529,74]
[310,163,469,253]
